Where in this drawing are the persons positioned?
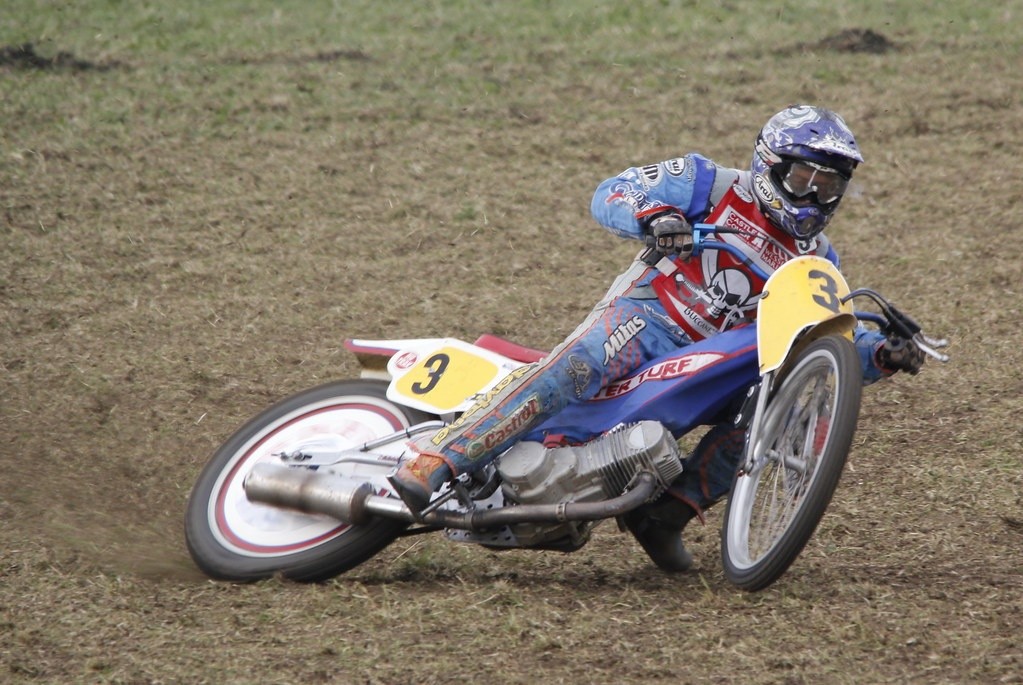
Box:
[386,104,925,577]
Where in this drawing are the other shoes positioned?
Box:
[622,503,694,573]
[385,453,452,512]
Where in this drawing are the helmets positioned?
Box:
[750,104,864,241]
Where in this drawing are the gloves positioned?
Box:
[879,337,926,376]
[648,212,694,262]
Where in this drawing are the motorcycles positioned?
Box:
[182,225,950,594]
